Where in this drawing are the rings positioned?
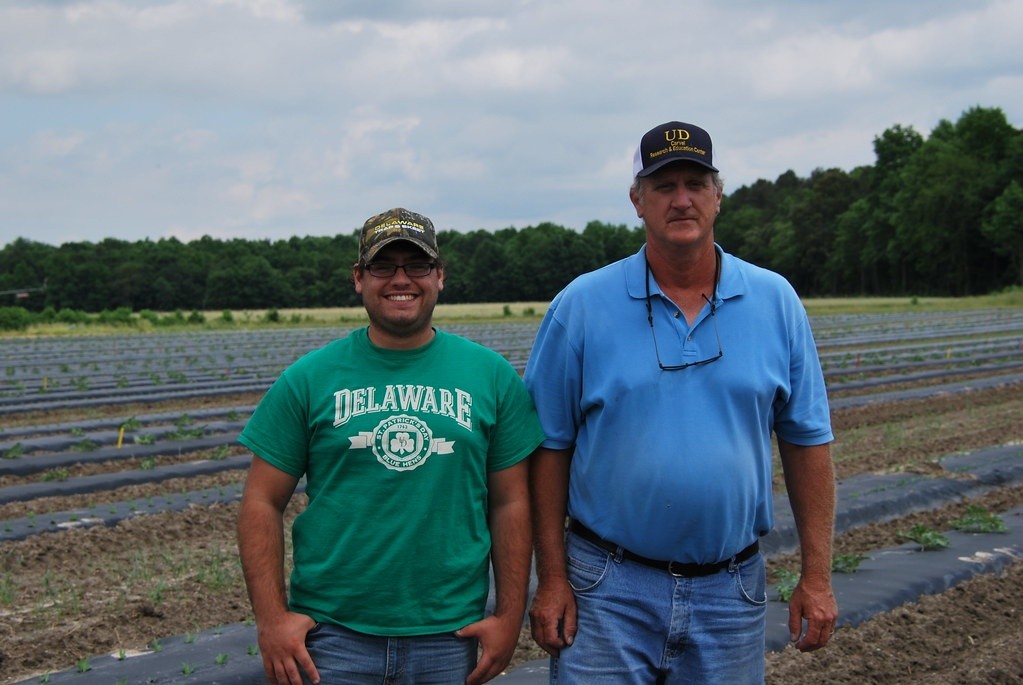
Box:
[829,630,834,635]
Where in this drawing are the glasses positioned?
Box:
[645,294,723,370]
[359,261,437,278]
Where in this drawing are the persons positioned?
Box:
[529,121,839,685]
[236,207,533,685]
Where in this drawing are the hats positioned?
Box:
[632,120,720,181]
[358,208,441,265]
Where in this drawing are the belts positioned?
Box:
[568,514,760,578]
